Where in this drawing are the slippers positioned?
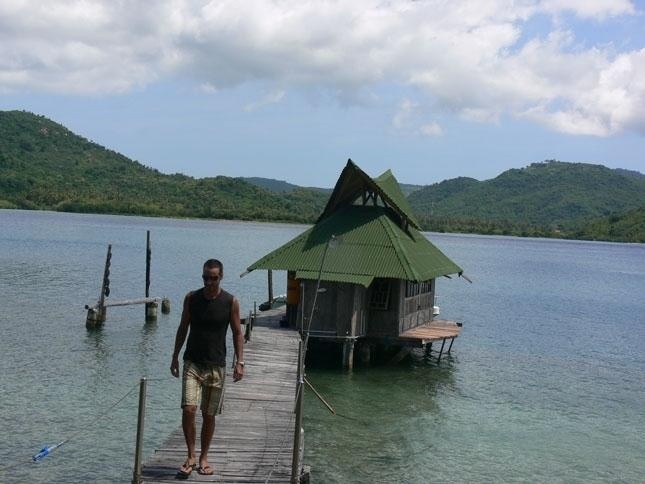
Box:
[199,465,213,475]
[178,460,196,475]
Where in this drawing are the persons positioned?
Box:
[286,272,299,330]
[170,259,246,477]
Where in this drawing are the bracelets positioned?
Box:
[237,361,245,366]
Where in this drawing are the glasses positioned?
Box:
[202,275,218,281]
